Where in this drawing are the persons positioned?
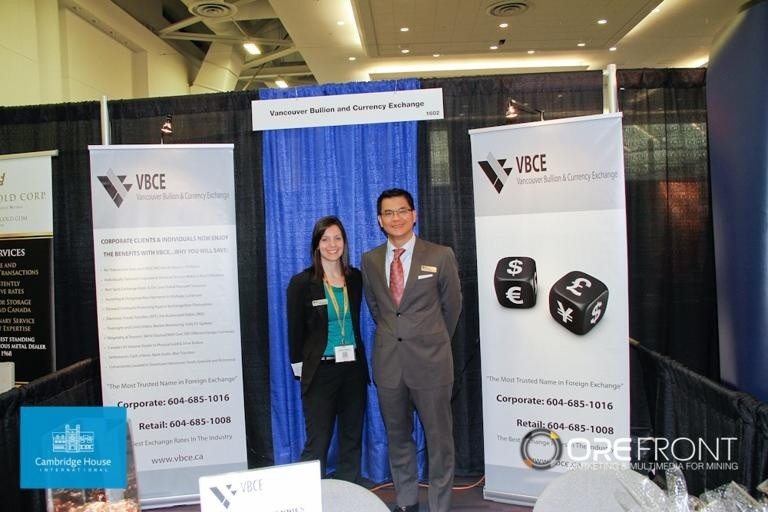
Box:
[286,216,362,484]
[360,188,463,512]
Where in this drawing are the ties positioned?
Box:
[389,248,406,307]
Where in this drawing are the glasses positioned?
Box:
[380,207,412,220]
